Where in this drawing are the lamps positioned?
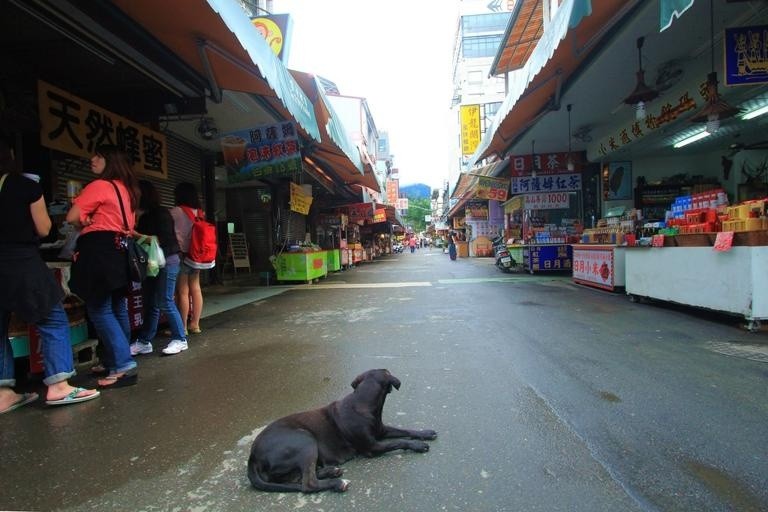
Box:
[622,36,658,123]
[690,0,743,132]
[532,140,537,179]
[567,105,575,170]
[196,112,217,140]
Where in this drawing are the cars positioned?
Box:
[392,243,399,250]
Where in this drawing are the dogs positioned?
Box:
[247,369,437,493]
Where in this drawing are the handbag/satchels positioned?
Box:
[127,236,148,283]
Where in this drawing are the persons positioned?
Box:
[67,144,147,388]
[460,231,466,242]
[0,133,100,414]
[393,236,428,255]
[454,232,459,242]
[165,183,219,336]
[129,177,189,356]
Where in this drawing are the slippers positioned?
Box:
[0,364,138,414]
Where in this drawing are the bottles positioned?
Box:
[745,209,762,231]
[582,210,637,248]
[528,216,577,246]
[637,179,729,239]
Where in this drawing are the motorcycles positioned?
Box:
[488,228,516,274]
[395,245,402,253]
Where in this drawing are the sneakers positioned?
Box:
[162,340,188,354]
[128,342,153,355]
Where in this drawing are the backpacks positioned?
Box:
[179,205,217,270]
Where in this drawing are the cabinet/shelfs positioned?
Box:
[570,244,625,292]
[340,248,367,267]
[624,246,768,332]
[328,249,340,272]
[507,244,524,266]
[525,244,573,274]
[9,257,93,373]
[275,250,328,283]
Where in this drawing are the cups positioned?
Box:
[220,138,248,175]
